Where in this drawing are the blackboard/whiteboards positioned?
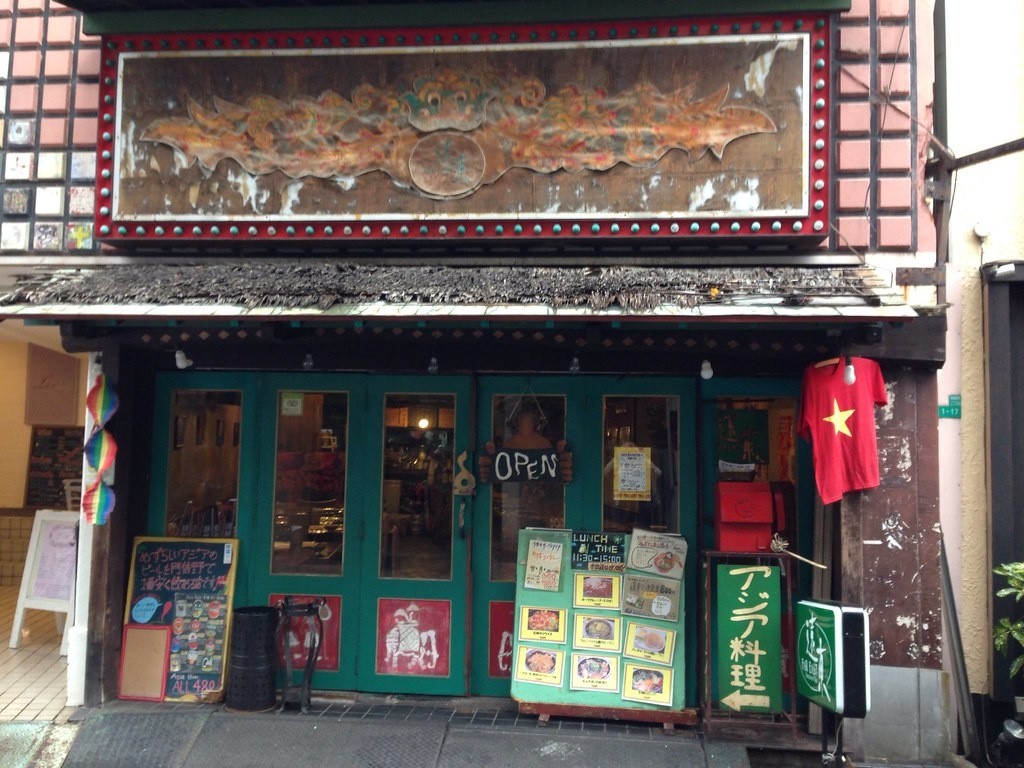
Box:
[119,537,240,705]
[22,423,85,510]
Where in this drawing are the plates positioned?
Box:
[633,669,663,694]
[579,657,612,679]
[585,619,612,638]
[526,650,555,672]
[636,626,665,652]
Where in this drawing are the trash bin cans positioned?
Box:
[223,606,278,712]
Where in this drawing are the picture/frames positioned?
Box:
[174,414,240,448]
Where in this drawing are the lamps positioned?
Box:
[990,719,1024,768]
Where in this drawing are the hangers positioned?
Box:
[814,335,840,369]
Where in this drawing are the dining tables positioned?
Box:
[169,491,677,574]
